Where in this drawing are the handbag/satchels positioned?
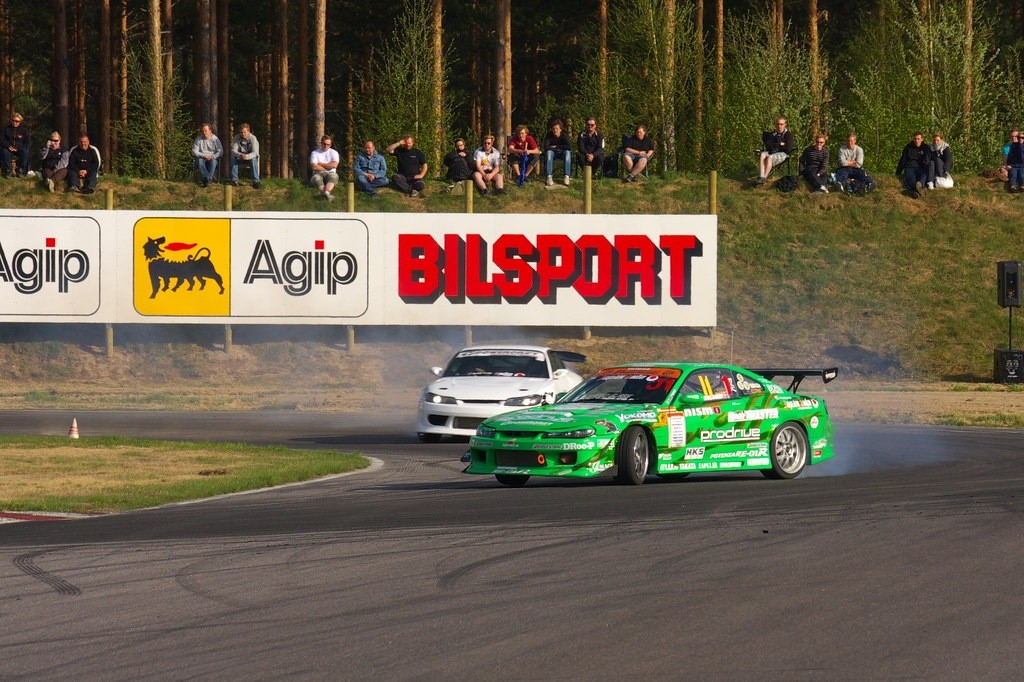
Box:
[850,177,876,196]
[777,175,798,192]
[935,172,953,189]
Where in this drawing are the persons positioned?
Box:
[797,134,829,194]
[472,135,505,197]
[1006,131,1024,193]
[192,123,223,188]
[443,138,477,183]
[353,140,389,197]
[836,131,877,193]
[755,116,792,188]
[310,134,339,203]
[507,124,540,183]
[640,376,666,403]
[67,131,101,194]
[926,131,951,190]
[998,131,1019,182]
[577,117,605,173]
[544,119,572,186]
[0,112,30,179]
[38,132,70,193]
[895,131,932,199]
[623,123,654,182]
[386,134,428,196]
[524,359,544,377]
[230,123,263,189]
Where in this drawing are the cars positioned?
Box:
[460,360,841,484]
[416,344,586,443]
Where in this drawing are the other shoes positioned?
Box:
[755,176,1024,197]
[322,192,335,202]
[627,174,637,183]
[253,182,260,189]
[201,177,210,188]
[231,180,239,186]
[476,172,601,197]
[46,178,55,193]
[83,187,94,194]
[69,185,80,193]
[411,189,419,196]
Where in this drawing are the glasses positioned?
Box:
[322,142,331,146]
[49,138,60,142]
[1017,136,1024,139]
[484,141,491,144]
[586,123,596,127]
[12,119,21,122]
[816,140,826,144]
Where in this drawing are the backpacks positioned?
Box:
[602,152,619,178]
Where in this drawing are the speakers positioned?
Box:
[994,347,1024,384]
[998,261,1021,308]
